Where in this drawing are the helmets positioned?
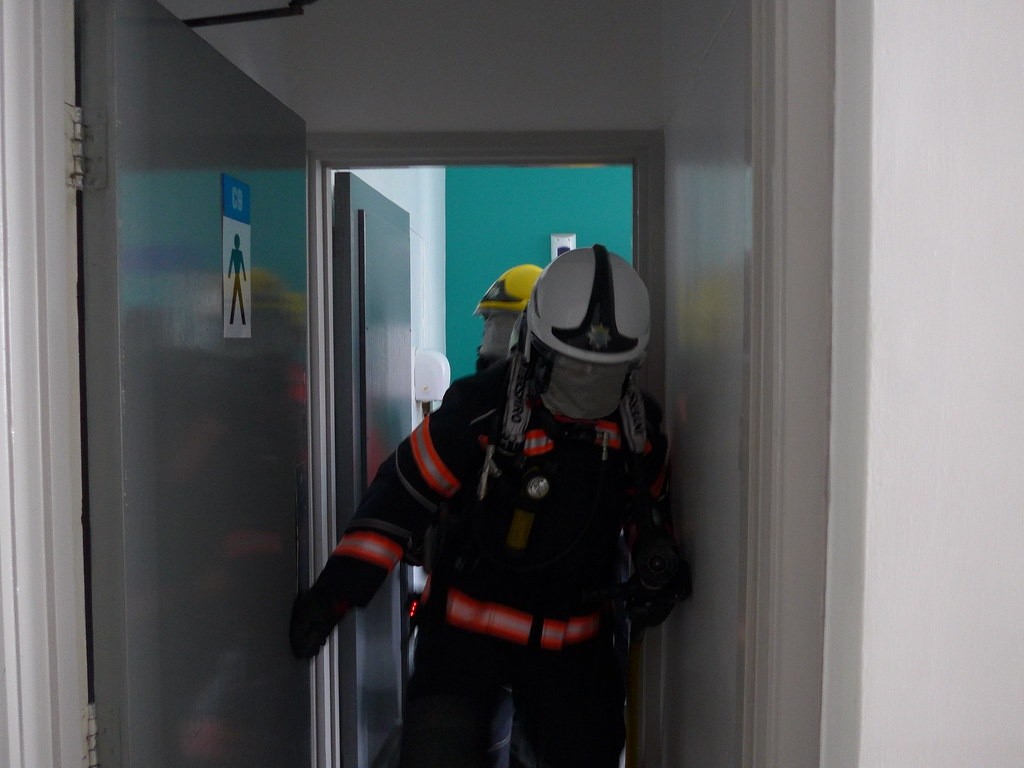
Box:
[528,246,651,363]
[472,264,544,317]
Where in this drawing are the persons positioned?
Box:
[288,245,697,768]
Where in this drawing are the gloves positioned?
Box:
[289,589,335,663]
[621,576,676,628]
